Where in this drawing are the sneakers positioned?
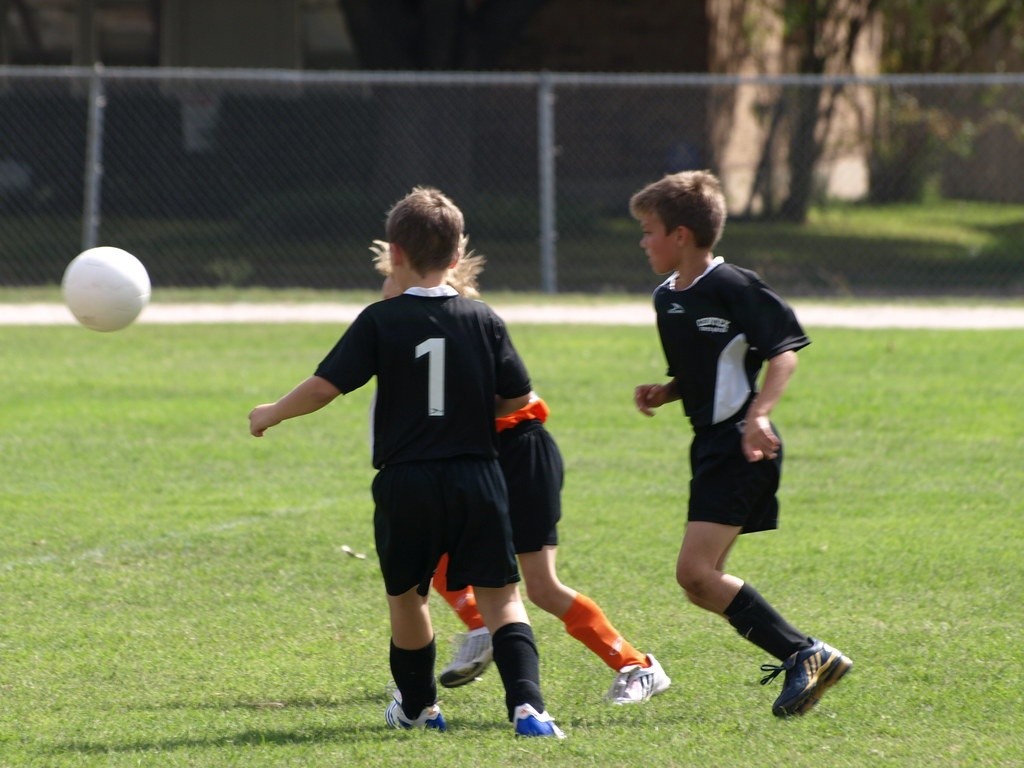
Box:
[760,636,842,718]
[438,627,496,689]
[382,678,447,734]
[592,653,671,705]
[797,656,855,718]
[511,704,567,741]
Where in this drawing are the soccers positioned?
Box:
[59,243,152,333]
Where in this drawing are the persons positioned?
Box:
[629,170,852,719]
[247,184,673,742]
